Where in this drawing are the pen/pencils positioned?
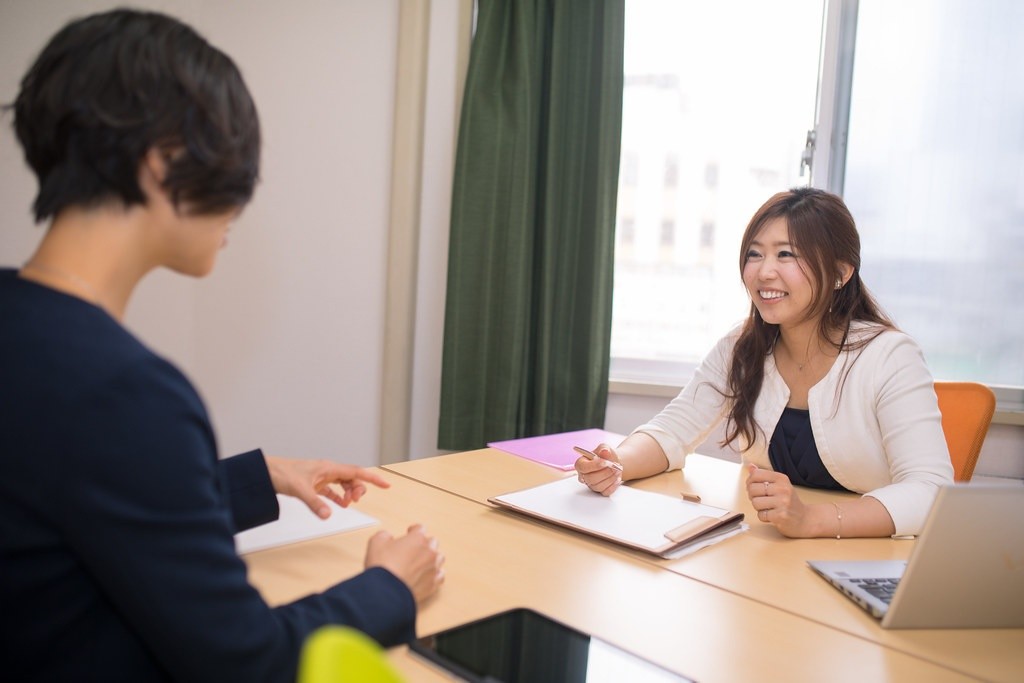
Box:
[573,446,623,472]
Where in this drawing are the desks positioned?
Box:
[379,446,1024,683]
[243,467,984,683]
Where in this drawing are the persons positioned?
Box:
[0,9,446,683]
[574,191,954,538]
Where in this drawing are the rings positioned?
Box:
[581,475,585,485]
[764,511,767,522]
[764,482,769,496]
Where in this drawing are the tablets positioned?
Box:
[409,606,697,683]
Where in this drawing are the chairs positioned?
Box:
[935,381,996,483]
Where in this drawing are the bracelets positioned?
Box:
[832,502,842,540]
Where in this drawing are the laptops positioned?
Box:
[808,483,1024,631]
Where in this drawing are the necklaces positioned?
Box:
[780,341,825,372]
